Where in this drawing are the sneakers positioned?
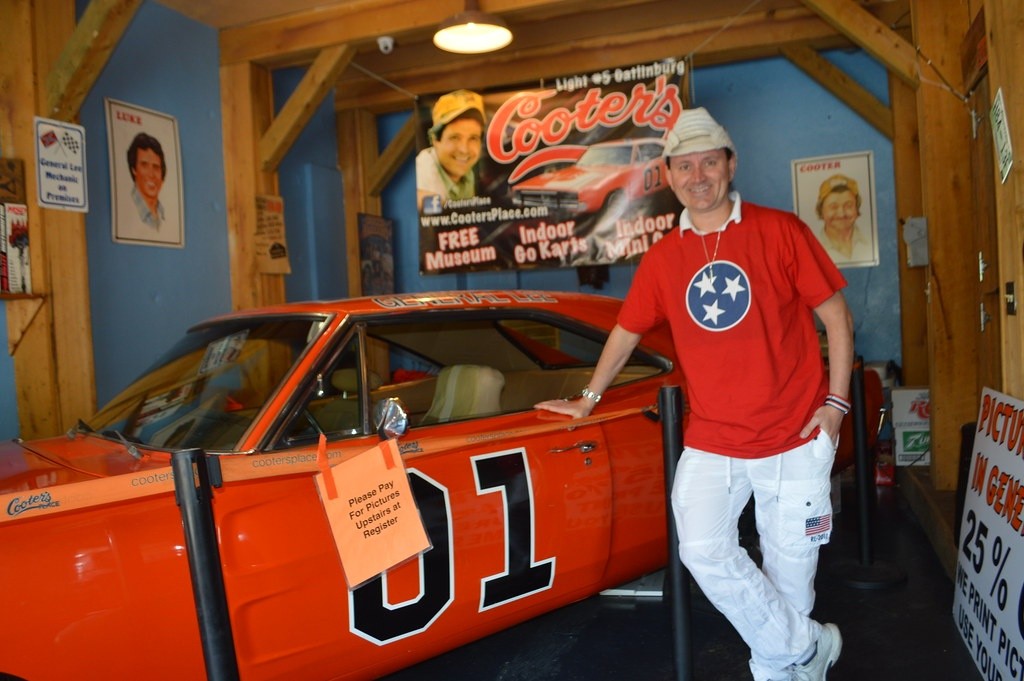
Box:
[769,622,842,681]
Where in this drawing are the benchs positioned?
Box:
[497,370,652,415]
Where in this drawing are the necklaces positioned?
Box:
[697,220,724,284]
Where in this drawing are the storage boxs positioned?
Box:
[890,384,931,466]
[864,361,901,439]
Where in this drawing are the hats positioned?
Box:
[431,89,487,126]
[818,175,860,202]
[661,106,739,160]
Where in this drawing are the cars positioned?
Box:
[512,139,675,215]
[0,288,886,681]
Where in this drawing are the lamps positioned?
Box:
[432,0,514,55]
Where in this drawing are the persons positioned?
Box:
[534,107,855,681]
[115,130,181,244]
[415,88,486,212]
[815,173,874,262]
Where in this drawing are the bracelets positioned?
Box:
[825,394,851,415]
[582,387,602,403]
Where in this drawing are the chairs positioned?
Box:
[418,363,506,425]
[310,369,382,437]
